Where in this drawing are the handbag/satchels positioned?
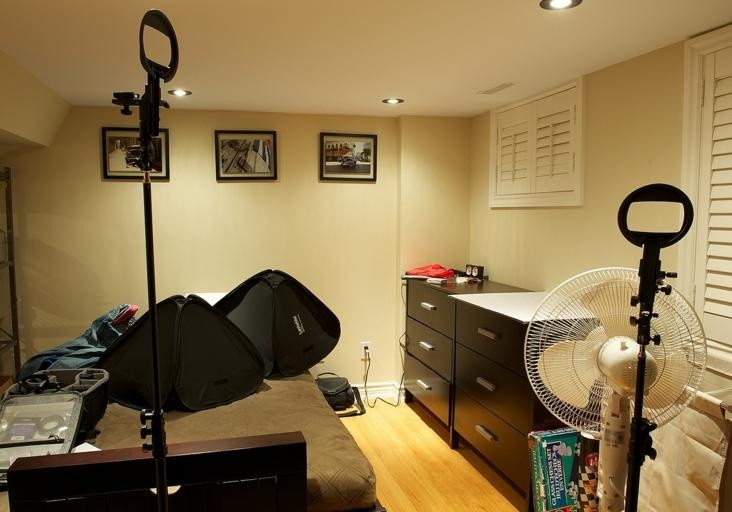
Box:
[311,372,366,418]
[16,304,139,383]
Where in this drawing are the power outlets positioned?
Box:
[360,340,372,361]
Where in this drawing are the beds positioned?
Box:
[0,357,387,511]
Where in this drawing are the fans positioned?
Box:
[522,264,710,510]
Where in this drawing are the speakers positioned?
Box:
[471,266,484,279]
[465,265,476,278]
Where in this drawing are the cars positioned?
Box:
[336,155,356,169]
[123,143,141,167]
[121,148,126,151]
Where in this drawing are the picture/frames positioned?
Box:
[101,126,170,182]
[213,128,279,182]
[318,130,379,182]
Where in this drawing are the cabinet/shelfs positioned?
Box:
[396,271,602,511]
